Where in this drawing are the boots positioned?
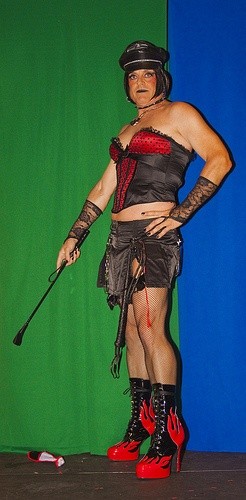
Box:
[107,377,157,462]
[136,384,186,479]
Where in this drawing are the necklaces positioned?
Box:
[129,97,166,127]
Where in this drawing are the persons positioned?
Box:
[55,40,232,479]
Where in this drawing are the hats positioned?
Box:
[119,40,169,71]
